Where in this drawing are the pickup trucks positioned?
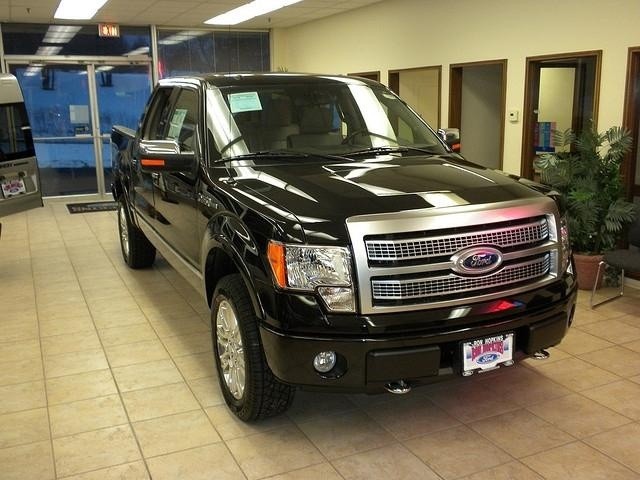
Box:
[112,71,580,424]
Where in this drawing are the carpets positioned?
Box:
[66,200,119,214]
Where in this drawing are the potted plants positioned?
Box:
[534,119,638,291]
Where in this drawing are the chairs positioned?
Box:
[231,112,266,153]
[590,210,640,309]
[260,101,299,149]
[287,108,345,148]
